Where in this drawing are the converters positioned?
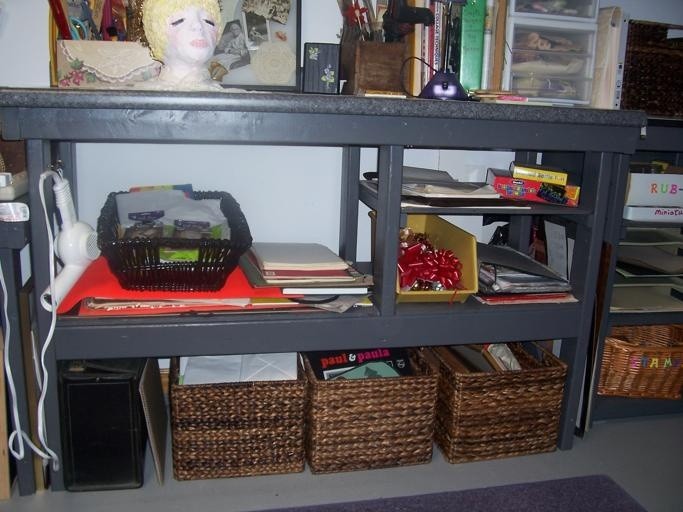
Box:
[0,172,12,188]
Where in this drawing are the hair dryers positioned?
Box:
[41,167,103,312]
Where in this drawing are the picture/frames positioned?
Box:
[211,0,304,93]
[48,1,149,88]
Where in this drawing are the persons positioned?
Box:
[134,0,225,90]
[224,23,250,69]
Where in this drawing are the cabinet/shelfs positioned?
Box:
[581,115,682,438]
[2,90,645,495]
[504,2,601,102]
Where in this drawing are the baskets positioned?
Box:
[434,343,568,465]
[619,19,683,120]
[301,348,441,475]
[169,340,569,482]
[597,325,683,400]
[170,352,309,481]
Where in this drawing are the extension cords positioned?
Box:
[0,168,29,201]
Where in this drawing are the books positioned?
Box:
[477,241,572,295]
[486,161,580,208]
[402,0,506,96]
[239,243,374,295]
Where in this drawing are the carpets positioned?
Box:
[254,473,656,512]
